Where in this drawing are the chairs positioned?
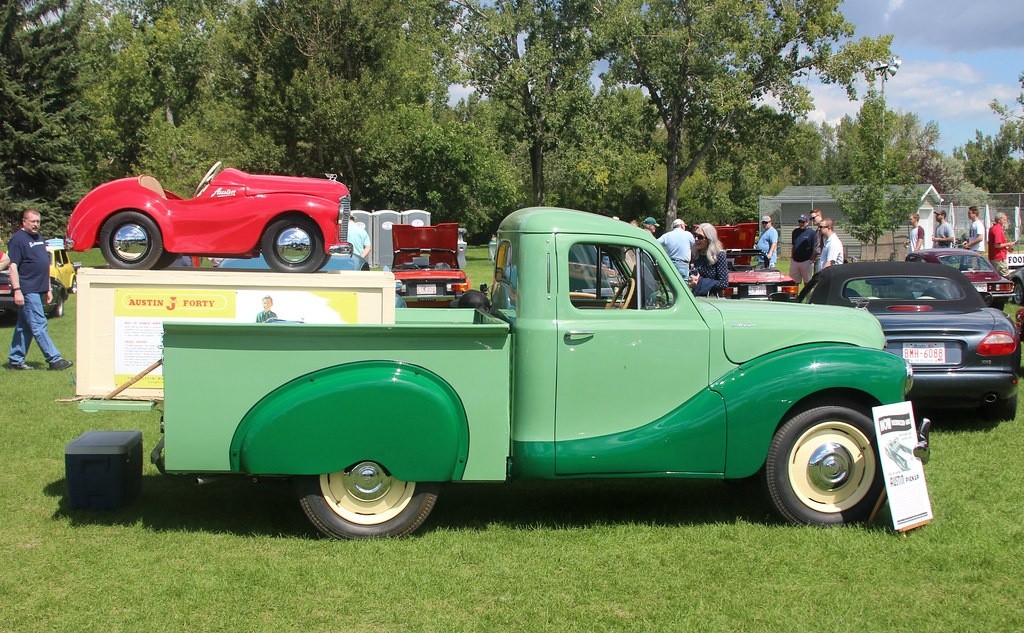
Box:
[138,174,166,198]
[921,286,948,299]
[845,288,861,297]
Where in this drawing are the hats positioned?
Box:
[761,216,771,222]
[934,210,946,217]
[797,214,809,222]
[644,217,660,227]
[671,219,687,227]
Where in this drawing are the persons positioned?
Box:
[8,209,73,370]
[932,210,954,248]
[569,217,729,298]
[908,213,925,252]
[789,210,844,298]
[755,216,778,268]
[0,251,10,270]
[347,211,371,259]
[988,213,1015,279]
[963,206,985,270]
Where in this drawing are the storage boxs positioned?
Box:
[65,430,143,510]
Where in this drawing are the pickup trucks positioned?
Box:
[77,207,933,543]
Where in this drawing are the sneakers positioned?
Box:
[49,358,73,370]
[6,363,33,371]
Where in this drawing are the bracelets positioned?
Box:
[14,288,21,291]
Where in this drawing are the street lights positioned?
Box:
[873,56,902,178]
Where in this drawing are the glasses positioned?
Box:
[693,234,707,240]
[810,215,818,220]
[818,225,830,229]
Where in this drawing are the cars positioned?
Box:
[63,159,355,273]
[0,267,68,324]
[46,246,78,294]
[691,221,799,304]
[213,251,407,308]
[904,248,1017,312]
[390,222,469,309]
[768,260,1021,422]
[1008,267,1024,306]
